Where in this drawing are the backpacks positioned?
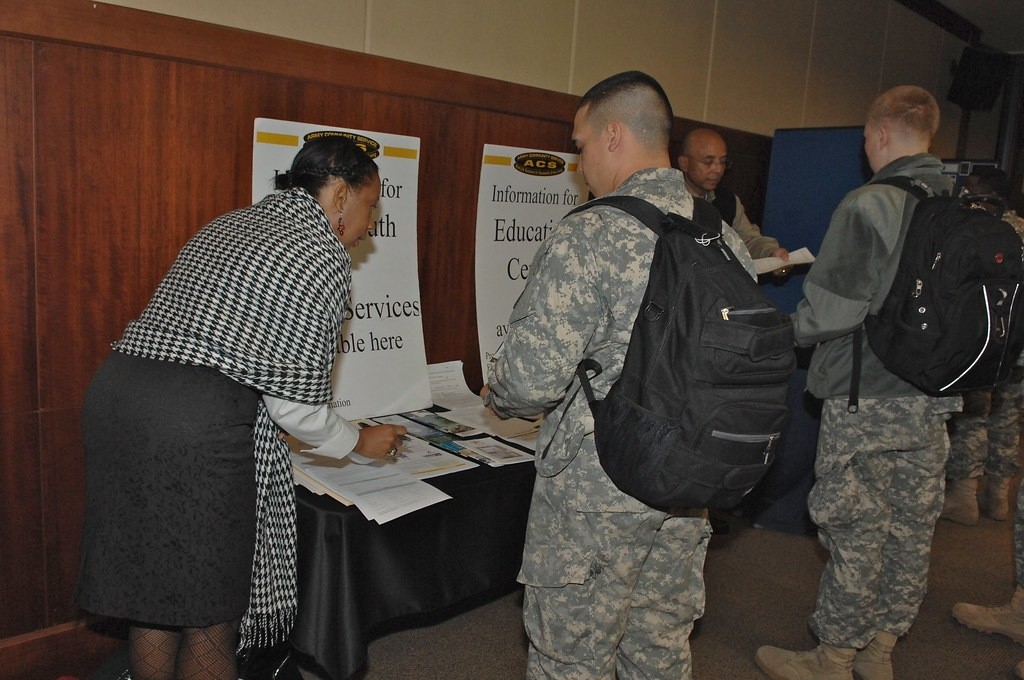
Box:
[560,196,796,514]
[846,175,1024,413]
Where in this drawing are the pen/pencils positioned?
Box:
[357,421,410,441]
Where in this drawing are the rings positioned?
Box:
[387,446,398,457]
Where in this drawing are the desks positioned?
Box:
[278,391,541,680]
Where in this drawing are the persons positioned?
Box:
[756,84,975,679]
[74,134,408,680]
[677,127,791,535]
[479,70,758,680]
[941,166,1022,526]
[951,476,1024,680]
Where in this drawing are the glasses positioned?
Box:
[687,155,733,172]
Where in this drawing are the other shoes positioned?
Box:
[852,630,900,679]
[938,478,980,526]
[755,642,856,679]
[952,583,1023,647]
[975,475,1012,521]
